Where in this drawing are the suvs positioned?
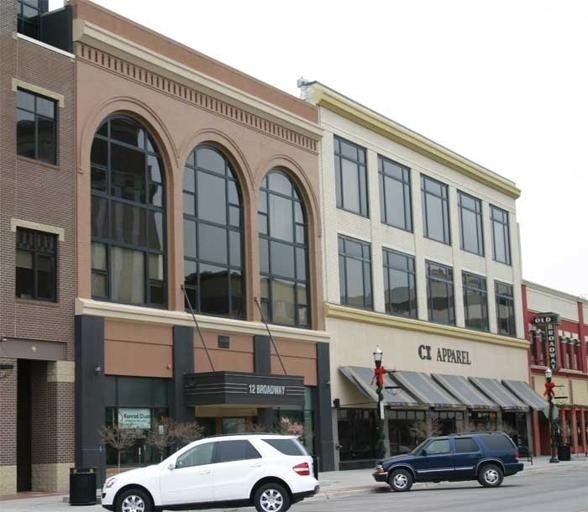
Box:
[101,435,319,511]
[372,432,523,491]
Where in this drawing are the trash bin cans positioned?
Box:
[558,442,570,460]
[70,466,97,506]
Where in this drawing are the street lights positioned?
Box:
[372,344,388,468]
[545,366,559,463]
